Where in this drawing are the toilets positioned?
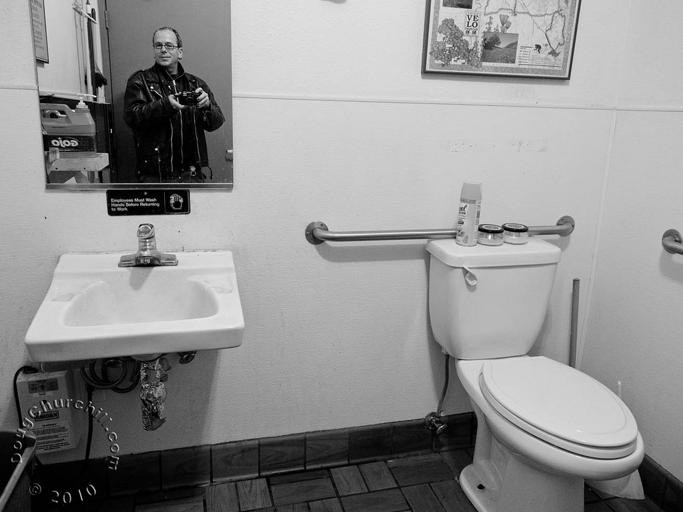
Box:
[425,236,644,512]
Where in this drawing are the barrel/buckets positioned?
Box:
[37,95,97,153]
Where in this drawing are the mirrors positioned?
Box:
[30,0,236,190]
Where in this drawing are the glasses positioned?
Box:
[156,44,179,49]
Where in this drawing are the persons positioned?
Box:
[122,26,226,182]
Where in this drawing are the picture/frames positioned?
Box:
[421,1,582,81]
[30,1,50,64]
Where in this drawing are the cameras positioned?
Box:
[177,92,203,106]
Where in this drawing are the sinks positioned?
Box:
[23,250,244,362]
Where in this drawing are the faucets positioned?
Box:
[117,223,178,267]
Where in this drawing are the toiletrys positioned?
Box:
[502,222,530,244]
[478,224,504,246]
